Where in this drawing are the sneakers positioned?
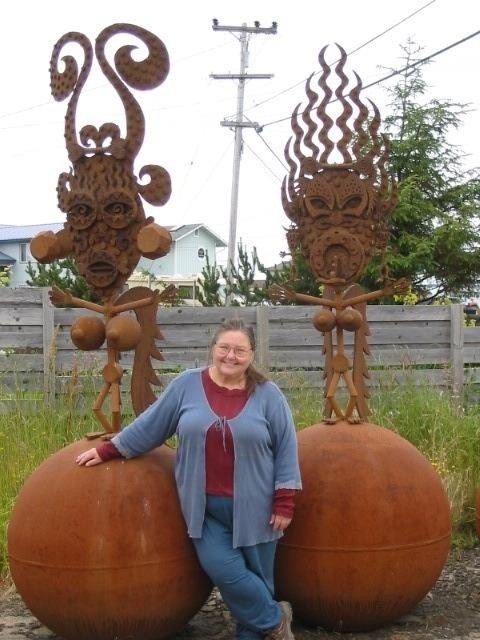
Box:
[264,600,295,640]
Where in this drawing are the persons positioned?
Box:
[75,317,302,640]
